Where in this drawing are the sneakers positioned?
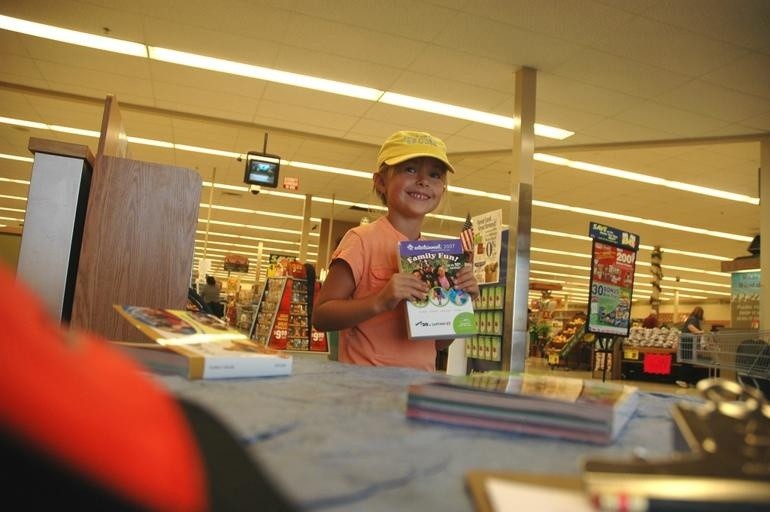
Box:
[675,379,697,390]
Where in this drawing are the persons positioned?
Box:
[674,305,705,388]
[642,309,658,328]
[311,130,480,374]
[200,276,222,319]
[525,308,537,361]
[413,259,455,305]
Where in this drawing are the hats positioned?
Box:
[376,130,456,175]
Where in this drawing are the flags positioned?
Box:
[459,219,473,253]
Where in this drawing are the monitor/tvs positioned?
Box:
[245,159,279,188]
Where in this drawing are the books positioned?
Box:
[106,303,294,382]
[395,237,480,340]
[405,369,640,446]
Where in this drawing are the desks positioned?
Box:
[140,341,704,511]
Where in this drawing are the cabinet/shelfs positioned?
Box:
[543,309,588,366]
[248,276,308,352]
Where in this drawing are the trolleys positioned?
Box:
[676,330,770,390]
